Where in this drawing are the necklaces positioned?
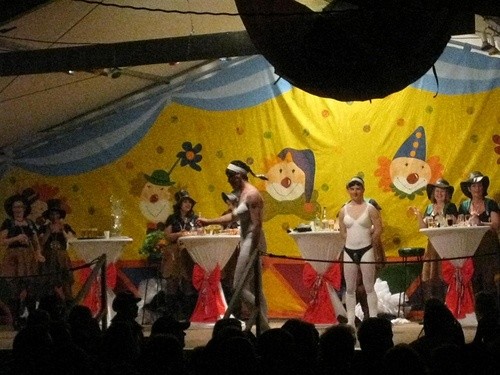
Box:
[472,199,483,210]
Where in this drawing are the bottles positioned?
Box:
[323,207,327,220]
[314,205,321,230]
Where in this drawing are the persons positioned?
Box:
[194,161,270,334]
[164,191,199,329]
[0,193,46,329]
[420,178,457,325]
[455,172,500,291]
[42,199,75,302]
[335,176,389,333]
[5,286,500,375]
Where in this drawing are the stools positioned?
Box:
[398,248,425,319]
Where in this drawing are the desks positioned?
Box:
[420,226,490,326]
[290,231,347,325]
[182,236,241,329]
[68,237,133,327]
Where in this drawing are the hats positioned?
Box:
[221,188,242,208]
[460,171,489,199]
[111,293,142,312]
[4,194,31,218]
[426,178,455,202]
[41,198,66,220]
[151,316,191,332]
[173,189,196,209]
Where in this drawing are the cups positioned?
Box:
[81,228,98,238]
[328,220,334,231]
[104,231,110,239]
[427,214,472,228]
[321,220,328,230]
